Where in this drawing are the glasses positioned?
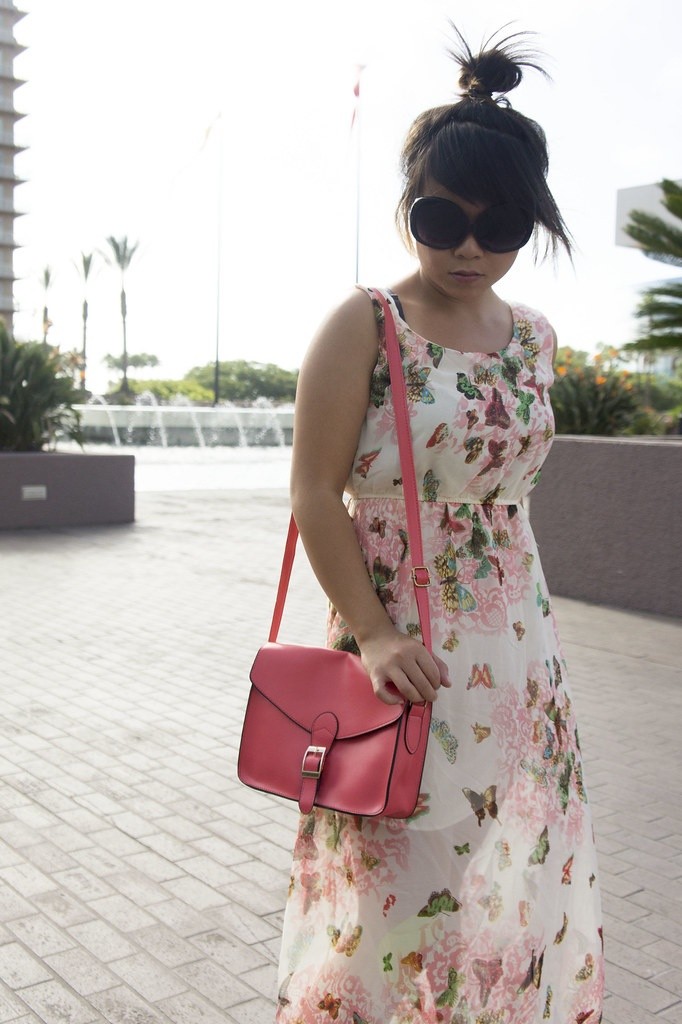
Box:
[409,196,535,253]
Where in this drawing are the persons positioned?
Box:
[274,49,604,1024]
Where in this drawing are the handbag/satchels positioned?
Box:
[236,640,431,820]
